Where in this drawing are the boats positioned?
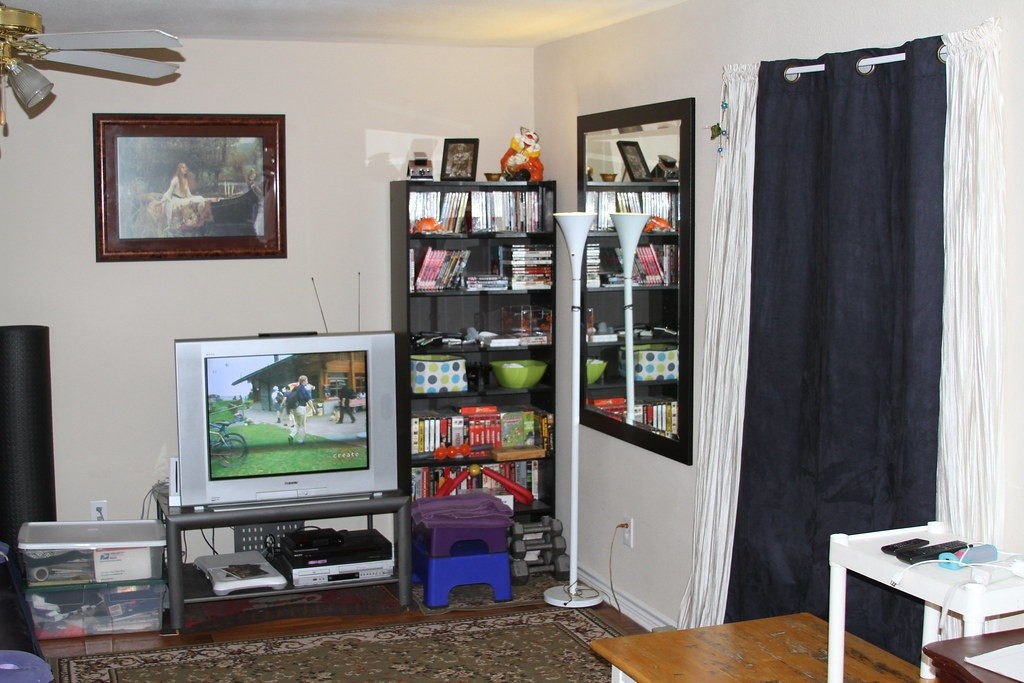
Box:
[136,180,263,233]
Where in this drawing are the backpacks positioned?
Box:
[275,392,282,405]
[287,386,300,409]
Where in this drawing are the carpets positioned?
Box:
[46,606,629,683]
[410,574,569,616]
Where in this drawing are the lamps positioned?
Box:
[543,212,604,608]
[4,56,55,109]
[609,212,650,424]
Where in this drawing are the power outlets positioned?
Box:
[623,517,633,548]
[90,500,108,521]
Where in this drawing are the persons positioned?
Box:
[272,386,295,428]
[288,375,316,445]
[160,163,205,230]
[335,380,356,424]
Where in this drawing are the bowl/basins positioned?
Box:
[490,360,547,389]
[484,173,501,182]
[586,357,608,385]
[601,174,618,182]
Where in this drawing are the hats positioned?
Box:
[273,386,279,390]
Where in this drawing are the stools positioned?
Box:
[412,495,513,608]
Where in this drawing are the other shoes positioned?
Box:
[351,418,356,423]
[283,423,287,426]
[288,436,293,446]
[277,418,281,423]
[336,420,343,424]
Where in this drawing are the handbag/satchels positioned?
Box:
[289,412,295,427]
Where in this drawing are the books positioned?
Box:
[585,190,676,233]
[409,191,468,234]
[614,244,676,286]
[511,244,553,290]
[466,276,508,290]
[586,243,601,289]
[412,460,538,503]
[411,403,554,461]
[471,191,538,233]
[409,247,470,291]
[589,394,678,434]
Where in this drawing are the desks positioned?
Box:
[827,521,1024,683]
[155,486,411,630]
[590,612,1024,683]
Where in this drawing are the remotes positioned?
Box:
[881,538,974,564]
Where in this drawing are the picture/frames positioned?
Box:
[441,138,479,181]
[617,141,653,182]
[93,112,287,262]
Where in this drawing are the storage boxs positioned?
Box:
[17,519,167,640]
[410,354,468,393]
[620,344,679,382]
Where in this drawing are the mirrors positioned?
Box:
[576,96,697,466]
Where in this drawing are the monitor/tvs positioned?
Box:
[174,330,398,514]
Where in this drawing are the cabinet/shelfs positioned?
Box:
[389,180,557,526]
[584,179,681,435]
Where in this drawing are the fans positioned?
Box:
[0,3,183,130]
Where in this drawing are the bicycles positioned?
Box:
[207,421,249,467]
